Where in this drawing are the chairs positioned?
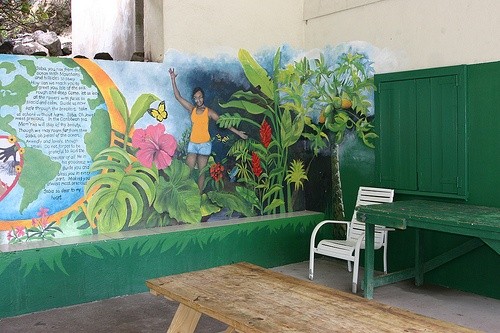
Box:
[308,186,394,296]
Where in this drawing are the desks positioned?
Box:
[354,200,500,300]
[145,262,491,333]
